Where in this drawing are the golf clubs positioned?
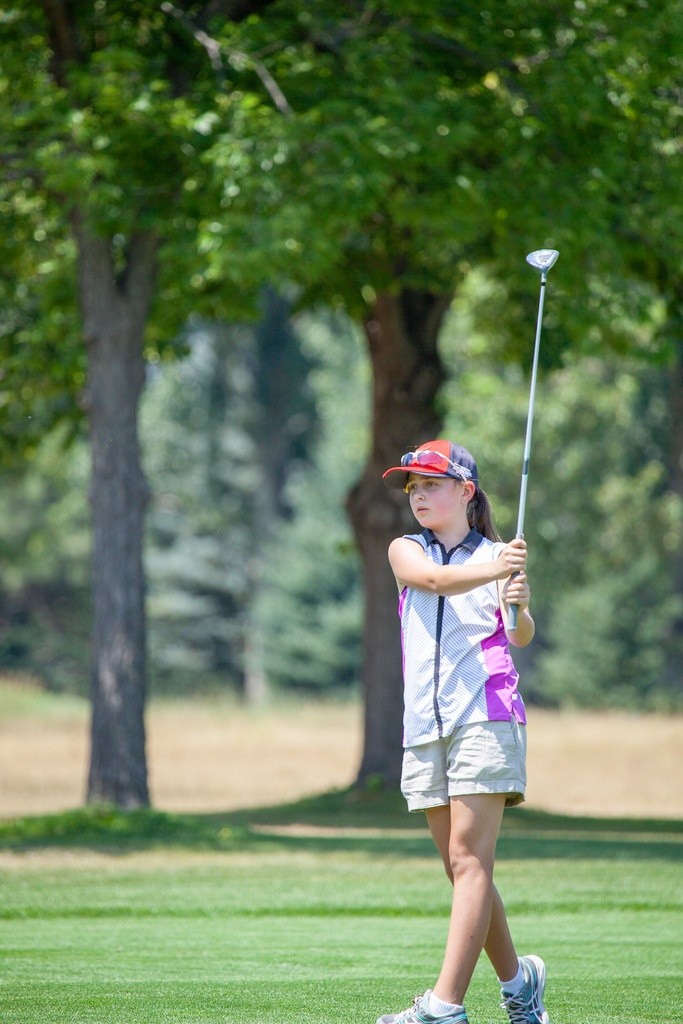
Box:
[505,247,560,632]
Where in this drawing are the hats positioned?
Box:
[382,440,478,493]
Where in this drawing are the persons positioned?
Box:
[371,439,551,1024]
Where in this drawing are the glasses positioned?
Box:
[400,450,471,482]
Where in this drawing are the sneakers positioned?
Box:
[498,954,550,1024]
[376,988,468,1024]
